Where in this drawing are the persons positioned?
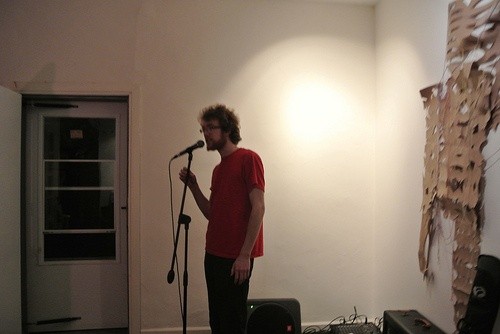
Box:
[179,106,265,334]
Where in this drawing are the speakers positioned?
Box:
[245,299,301,334]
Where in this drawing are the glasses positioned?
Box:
[200,126,222,133]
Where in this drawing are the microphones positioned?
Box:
[174,140,204,158]
